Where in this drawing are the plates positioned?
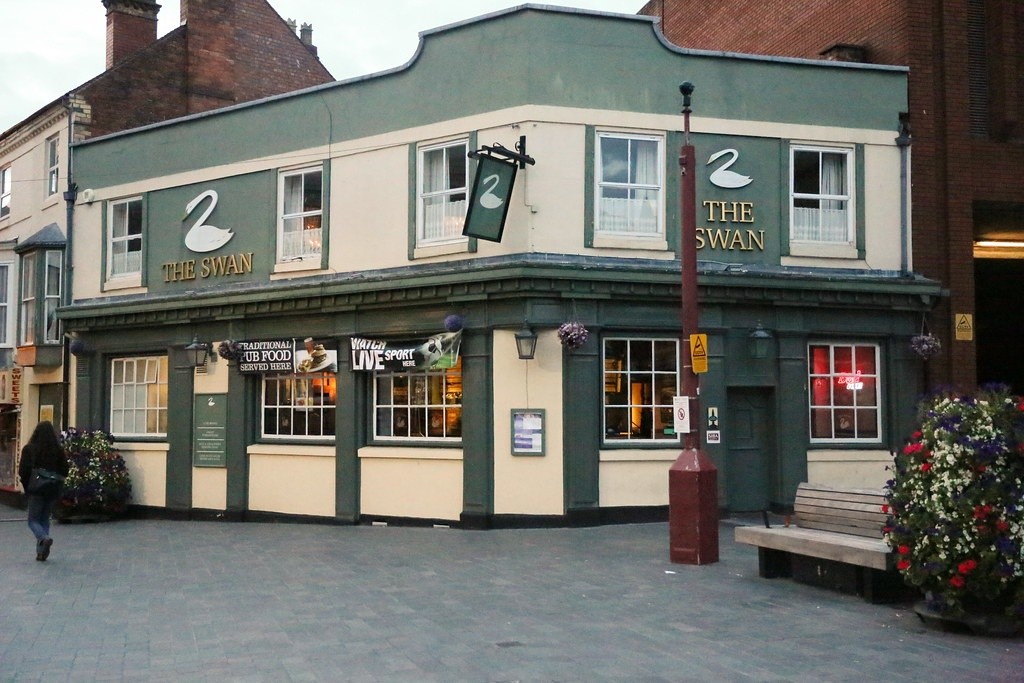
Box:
[297,355,333,372]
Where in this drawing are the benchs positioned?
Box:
[737,482,897,602]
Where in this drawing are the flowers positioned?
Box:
[909,335,943,360]
[53,427,130,515]
[219,339,245,360]
[876,382,1024,613]
[556,321,589,348]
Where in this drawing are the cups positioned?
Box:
[304,337,318,354]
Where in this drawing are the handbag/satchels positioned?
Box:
[28,469,66,501]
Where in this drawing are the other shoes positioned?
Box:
[36,537,53,561]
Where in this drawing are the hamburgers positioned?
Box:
[299,344,327,371]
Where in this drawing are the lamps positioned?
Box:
[185,291,197,296]
[513,319,539,359]
[69,339,96,357]
[747,319,773,360]
[724,263,742,273]
[184,333,213,368]
[974,240,1024,248]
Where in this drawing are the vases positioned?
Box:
[913,599,1024,639]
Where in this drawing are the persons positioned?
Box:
[18,421,69,561]
